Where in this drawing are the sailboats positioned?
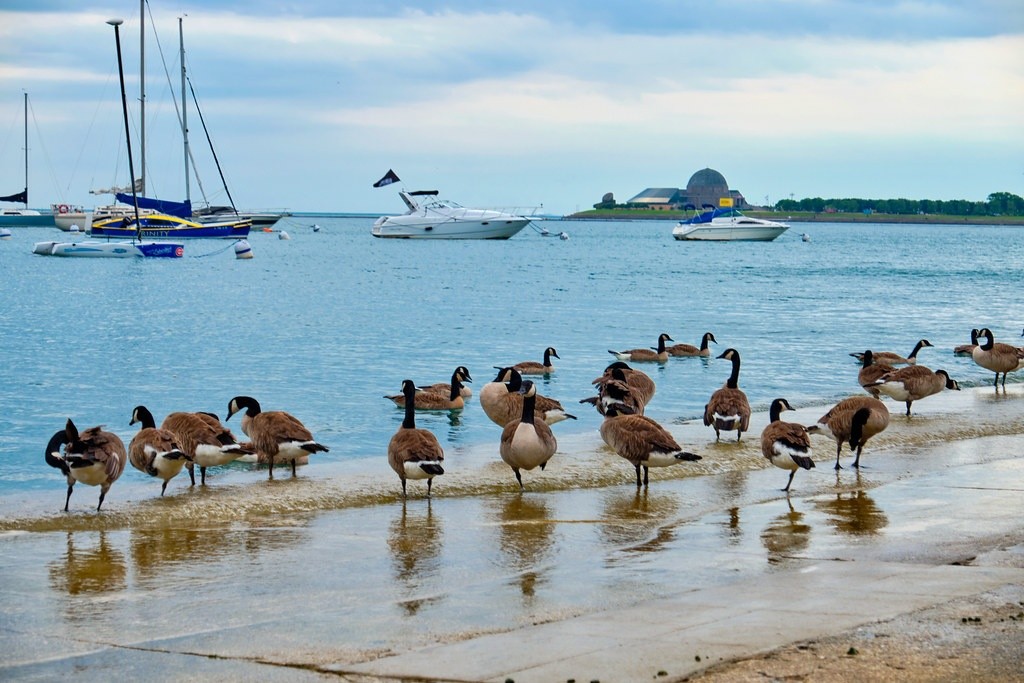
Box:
[0,0,326,264]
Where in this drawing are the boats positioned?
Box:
[371,167,572,243]
[671,198,790,242]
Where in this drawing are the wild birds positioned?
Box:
[859,339,935,366]
[953,329,980,353]
[500,381,558,491]
[388,380,445,496]
[759,398,816,492]
[863,365,961,415]
[649,333,718,357]
[817,396,890,471]
[400,366,473,397]
[127,406,194,497]
[225,396,330,480]
[972,328,1024,387]
[479,366,578,428]
[225,441,309,465]
[159,410,255,487]
[579,361,703,487]
[511,347,560,374]
[44,418,127,512]
[608,333,674,363]
[857,350,900,401]
[703,348,751,442]
[382,371,472,409]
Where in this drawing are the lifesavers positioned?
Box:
[59,204,68,214]
